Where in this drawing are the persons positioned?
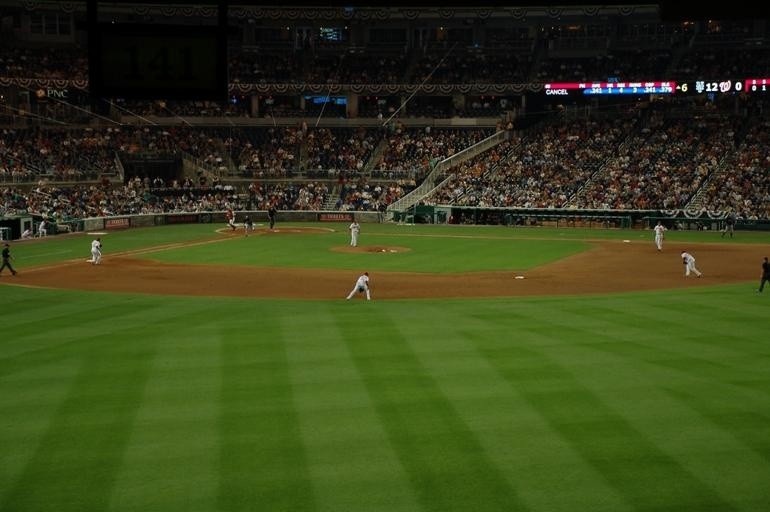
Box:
[22,227,32,239]
[242,215,255,237]
[757,256,770,292]
[86,237,102,265]
[680,250,702,278]
[653,220,669,251]
[720,209,736,240]
[39,217,51,238]
[347,219,360,247]
[1,2,769,231]
[345,271,372,302]
[0,244,17,276]
